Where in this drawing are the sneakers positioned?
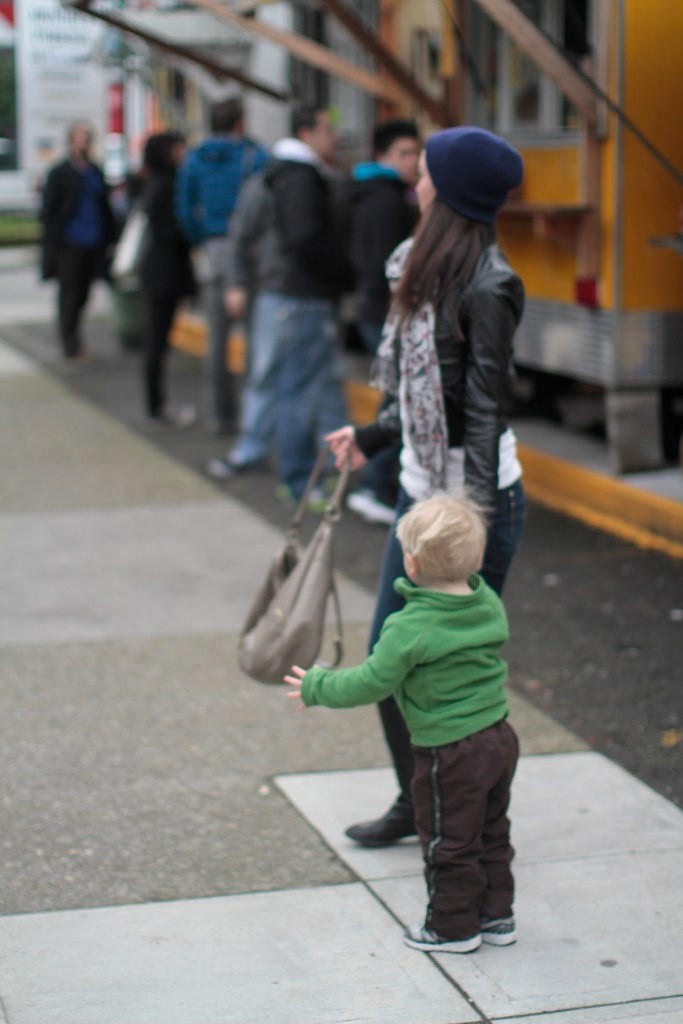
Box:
[478,919,517,946]
[403,924,481,953]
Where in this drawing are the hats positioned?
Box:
[427,126,523,224]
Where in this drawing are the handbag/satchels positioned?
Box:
[239,437,354,686]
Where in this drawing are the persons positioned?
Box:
[40,120,124,358]
[211,173,279,475]
[266,99,351,513]
[102,130,197,425]
[342,118,424,523]
[322,126,527,846]
[284,490,518,953]
[175,98,279,439]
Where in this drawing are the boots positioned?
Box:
[345,698,421,845]
